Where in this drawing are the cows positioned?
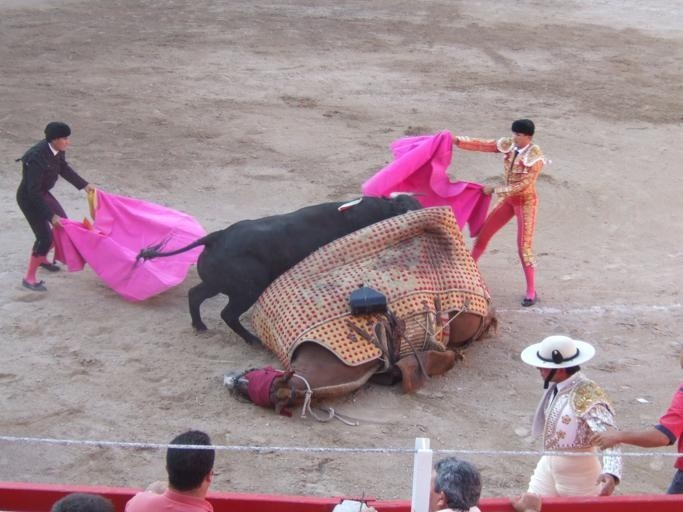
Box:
[135,193,428,346]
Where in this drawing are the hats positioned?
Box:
[520,334,597,370]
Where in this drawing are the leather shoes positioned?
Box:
[521,290,538,307]
[21,277,48,291]
[38,260,60,271]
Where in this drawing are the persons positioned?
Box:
[124,431,213,512]
[51,493,117,512]
[589,345,683,496]
[15,123,97,291]
[518,336,621,498]
[430,457,483,512]
[452,118,543,306]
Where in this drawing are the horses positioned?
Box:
[223,305,498,416]
